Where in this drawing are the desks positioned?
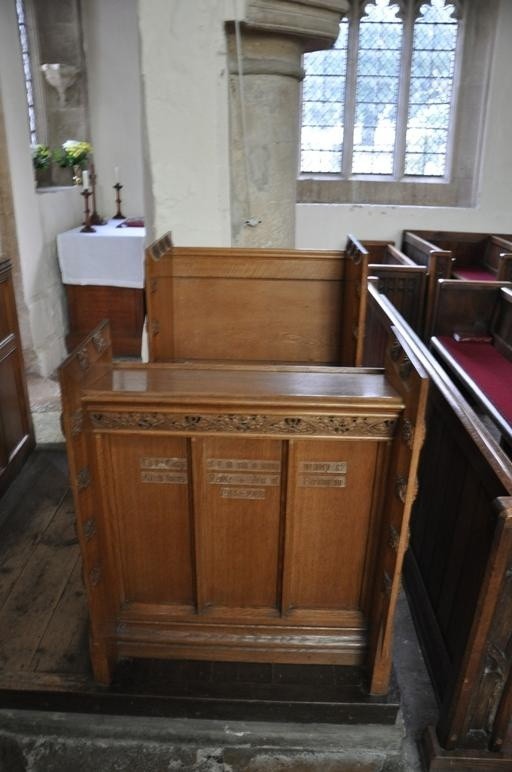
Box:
[56,218,146,356]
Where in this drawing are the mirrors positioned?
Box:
[15,0,92,191]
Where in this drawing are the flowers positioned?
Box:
[53,138,91,169]
[30,142,53,171]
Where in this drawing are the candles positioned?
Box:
[82,170,89,190]
[114,164,121,182]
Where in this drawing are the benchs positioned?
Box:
[352,228,512,450]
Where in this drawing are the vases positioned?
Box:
[73,165,81,181]
